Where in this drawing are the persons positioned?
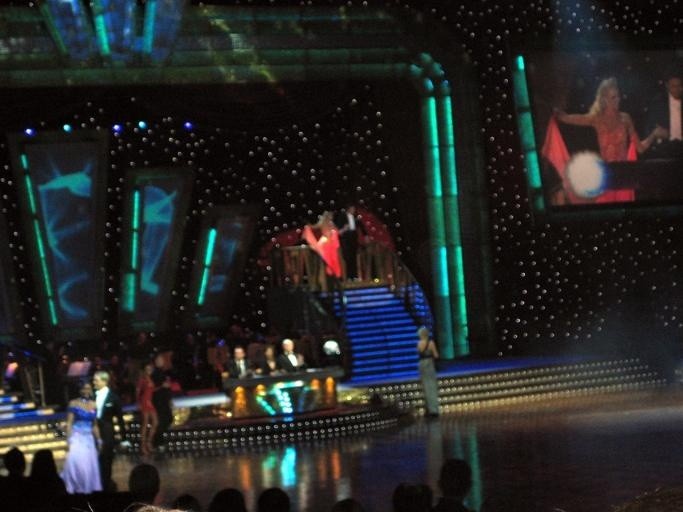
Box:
[418,327,439,418]
[553,76,683,203]
[0,331,472,511]
[307,204,370,281]
